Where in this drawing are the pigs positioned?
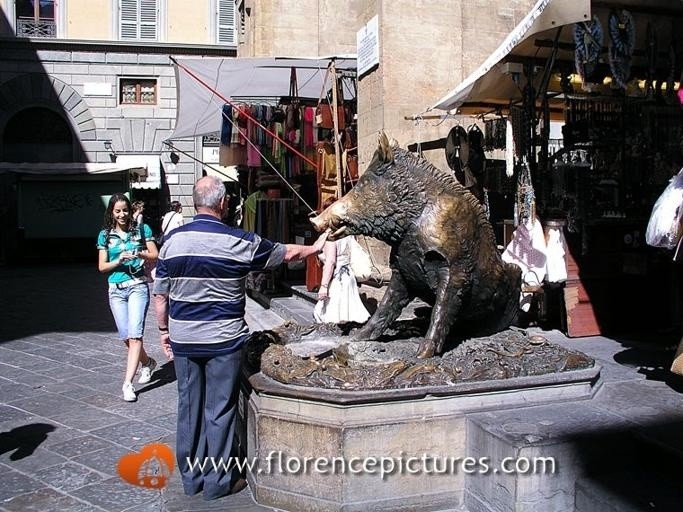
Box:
[308,130,523,362]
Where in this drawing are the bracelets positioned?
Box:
[320,284,329,288]
[158,327,167,330]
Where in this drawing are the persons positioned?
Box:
[152,176,332,500]
[313,196,374,336]
[132,199,146,223]
[161,201,184,239]
[96,192,159,403]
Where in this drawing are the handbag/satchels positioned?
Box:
[140,248,155,283]
[350,237,372,283]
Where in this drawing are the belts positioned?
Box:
[108,275,148,290]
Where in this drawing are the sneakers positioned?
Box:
[225,478,247,494]
[138,358,157,384]
[122,382,137,401]
